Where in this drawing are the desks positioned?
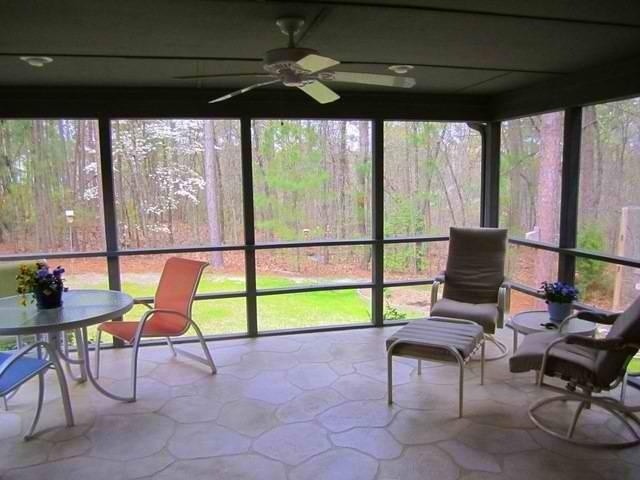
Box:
[0,290,134,384]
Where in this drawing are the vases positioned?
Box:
[40,292,63,309]
[547,302,572,321]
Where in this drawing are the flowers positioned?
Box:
[16,259,70,306]
[536,280,580,303]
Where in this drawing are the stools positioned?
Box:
[386,317,486,419]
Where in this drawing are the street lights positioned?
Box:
[62,209,79,273]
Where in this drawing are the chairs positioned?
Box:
[508,295,639,449]
[92,257,216,400]
[0,342,75,442]
[430,227,510,362]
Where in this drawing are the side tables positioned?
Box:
[512,311,596,352]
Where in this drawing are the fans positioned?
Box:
[170,17,417,105]
